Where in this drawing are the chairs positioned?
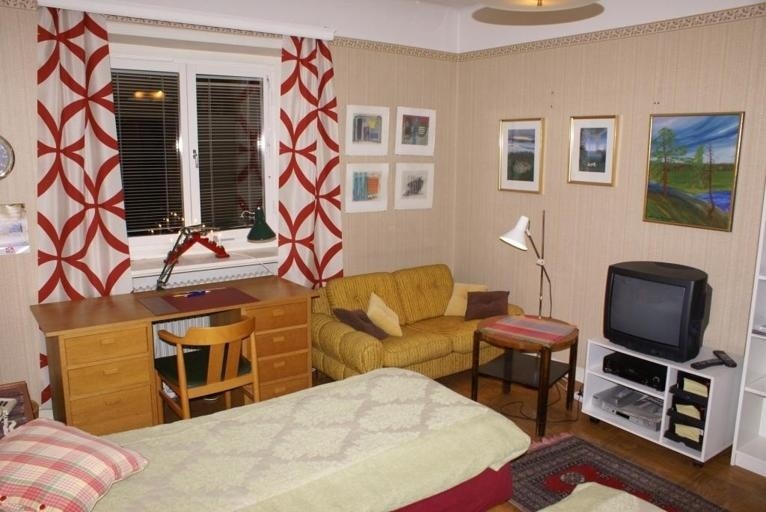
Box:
[155,315,260,420]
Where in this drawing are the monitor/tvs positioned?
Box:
[603,261,712,363]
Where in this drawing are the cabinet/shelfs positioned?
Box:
[730,183,766,479]
[30,276,321,435]
[581,339,739,468]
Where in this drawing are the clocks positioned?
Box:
[0,136,15,180]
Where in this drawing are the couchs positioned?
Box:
[311,264,524,382]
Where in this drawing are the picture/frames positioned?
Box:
[641,112,746,233]
[497,118,544,195]
[343,105,437,214]
[567,115,620,186]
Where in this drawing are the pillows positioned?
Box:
[0,417,148,512]
[326,264,510,339]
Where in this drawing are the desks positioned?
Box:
[0,381,35,436]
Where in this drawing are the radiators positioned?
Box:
[152,315,212,398]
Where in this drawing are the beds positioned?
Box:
[91,367,531,512]
[534,481,668,512]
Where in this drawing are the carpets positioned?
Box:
[505,432,730,512]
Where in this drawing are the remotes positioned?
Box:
[691,359,724,370]
[714,351,737,366]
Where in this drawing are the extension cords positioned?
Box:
[574,392,583,403]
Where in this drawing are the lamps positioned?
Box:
[500,216,554,319]
[240,206,275,241]
